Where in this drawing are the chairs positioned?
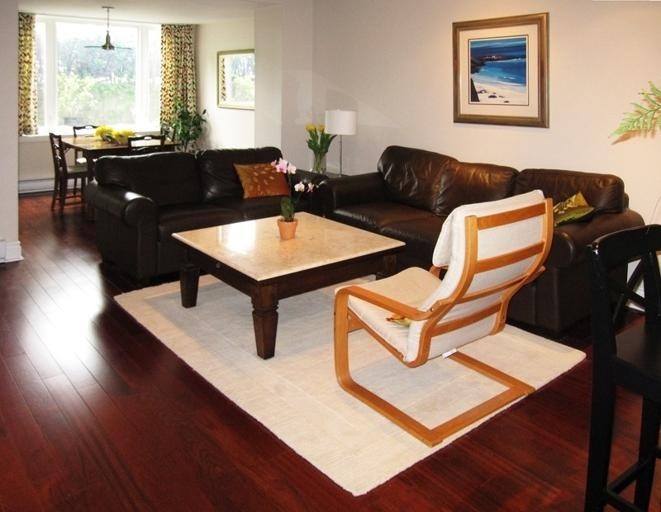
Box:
[331,187,556,449]
[48,132,97,217]
[126,133,166,156]
[582,222,660,511]
[72,123,101,196]
[159,121,177,143]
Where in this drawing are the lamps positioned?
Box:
[321,108,357,180]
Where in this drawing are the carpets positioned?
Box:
[112,272,588,498]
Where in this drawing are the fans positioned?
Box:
[83,4,133,51]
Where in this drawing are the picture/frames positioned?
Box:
[215,48,255,112]
[451,10,551,130]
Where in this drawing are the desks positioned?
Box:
[60,134,181,184]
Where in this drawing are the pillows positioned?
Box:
[232,162,291,200]
[551,188,592,229]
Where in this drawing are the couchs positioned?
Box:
[82,145,327,283]
[318,142,646,343]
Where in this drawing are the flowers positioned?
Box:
[94,126,136,145]
[268,156,317,220]
[302,122,336,172]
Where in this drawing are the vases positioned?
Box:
[309,152,329,175]
[275,219,299,239]
